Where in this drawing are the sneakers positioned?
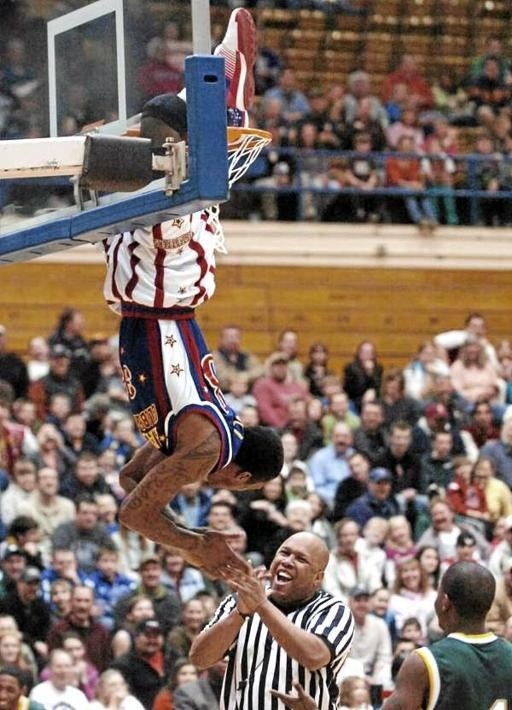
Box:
[213,8,258,146]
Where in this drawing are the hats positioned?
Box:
[46,343,74,359]
[138,617,165,635]
[84,331,109,345]
[0,537,29,564]
[138,552,161,568]
[23,566,40,582]
[82,393,109,414]
[267,351,291,367]
[425,402,446,418]
[348,584,368,597]
[367,467,393,482]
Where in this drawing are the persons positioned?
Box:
[1,1,512,708]
